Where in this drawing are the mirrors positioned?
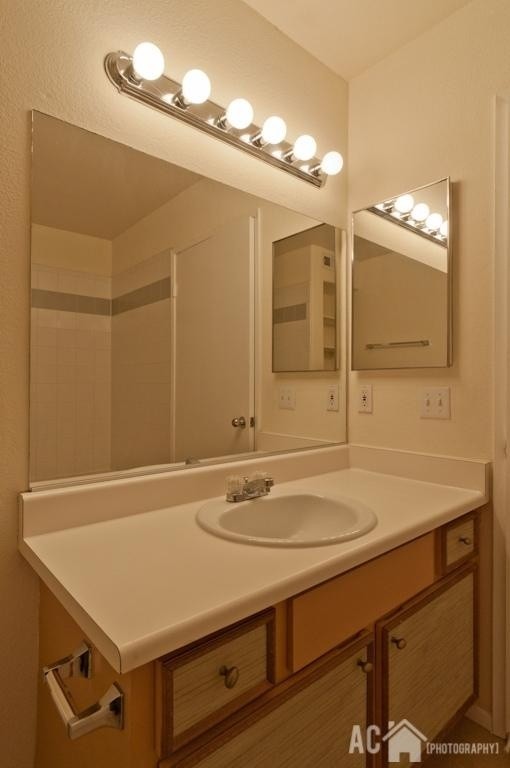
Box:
[26,108,349,485]
[269,222,339,374]
[351,175,455,372]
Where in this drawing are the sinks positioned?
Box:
[196,488,377,548]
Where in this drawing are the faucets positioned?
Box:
[186,457,200,466]
[226,472,274,502]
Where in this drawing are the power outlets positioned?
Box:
[326,384,340,411]
[357,385,373,413]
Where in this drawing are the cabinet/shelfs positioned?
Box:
[33,509,482,767]
